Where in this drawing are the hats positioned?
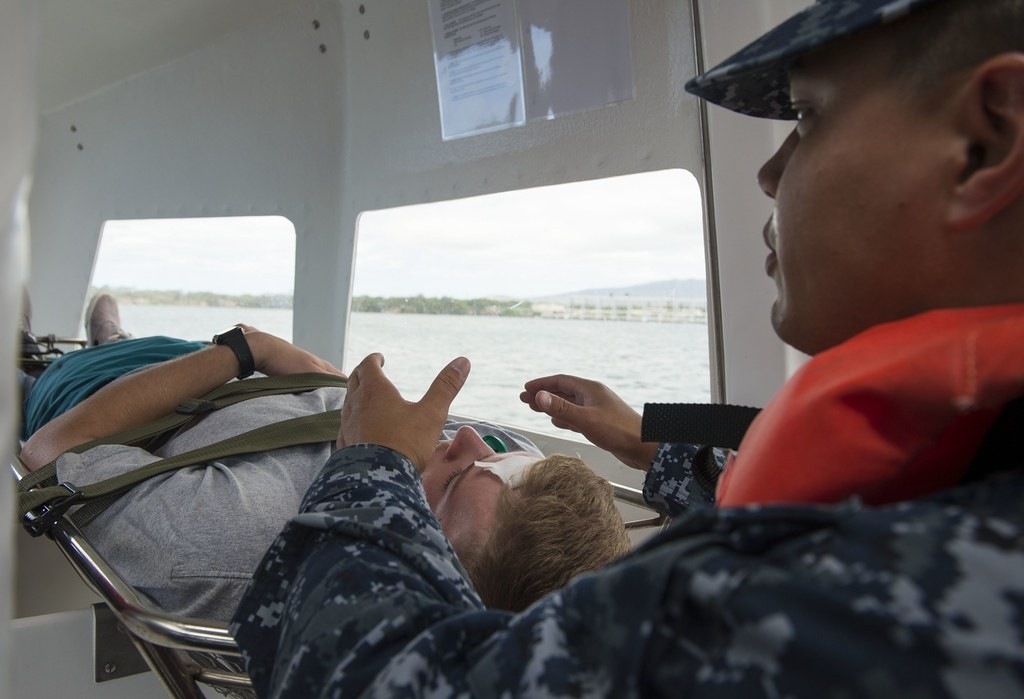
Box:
[683,0,919,120]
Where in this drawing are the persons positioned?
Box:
[233,0,1024,699]
[18,287,631,624]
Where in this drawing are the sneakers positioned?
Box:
[84,293,132,348]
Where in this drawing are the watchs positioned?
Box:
[212,326,255,380]
